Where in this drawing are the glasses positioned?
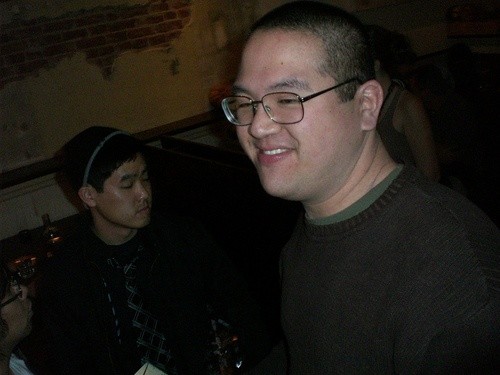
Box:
[0,278,23,309]
[220,75,362,126]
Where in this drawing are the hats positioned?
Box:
[58,124,132,210]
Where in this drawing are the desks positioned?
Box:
[0,209,93,375]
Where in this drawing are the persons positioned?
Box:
[364,24,439,181]
[0,124,269,375]
[220,1,500,375]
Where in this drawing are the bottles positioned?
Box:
[210,317,241,371]
[41,211,63,247]
